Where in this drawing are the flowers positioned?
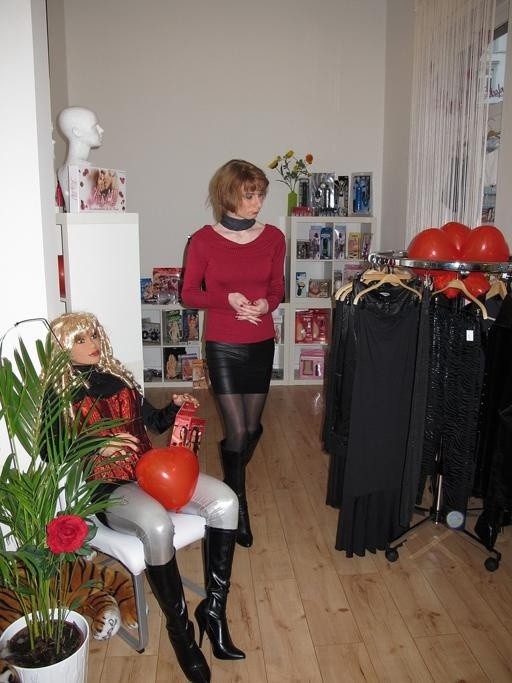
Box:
[267,150,314,189]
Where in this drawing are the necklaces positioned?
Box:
[220,213,256,230]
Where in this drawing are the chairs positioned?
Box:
[54,455,213,654]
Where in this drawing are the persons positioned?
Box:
[41,312,248,682]
[89,167,105,195]
[178,425,187,446]
[101,168,118,191]
[186,427,201,457]
[180,159,287,548]
[166,352,178,378]
[54,105,104,210]
[167,319,180,342]
[183,358,192,378]
[187,313,199,339]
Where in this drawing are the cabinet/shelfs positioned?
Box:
[284,215,378,385]
[141,300,290,387]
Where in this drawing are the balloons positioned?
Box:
[406,228,458,298]
[439,220,470,252]
[135,446,202,513]
[458,223,508,301]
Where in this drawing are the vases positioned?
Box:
[287,189,298,210]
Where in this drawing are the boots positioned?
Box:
[220,427,263,548]
[144,525,246,683]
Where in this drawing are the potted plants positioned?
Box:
[1,336,145,683]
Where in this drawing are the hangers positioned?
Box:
[335,252,512,321]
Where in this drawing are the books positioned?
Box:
[176,352,198,381]
[191,358,210,389]
[182,308,200,342]
[162,308,184,345]
[141,317,161,345]
[162,346,187,380]
